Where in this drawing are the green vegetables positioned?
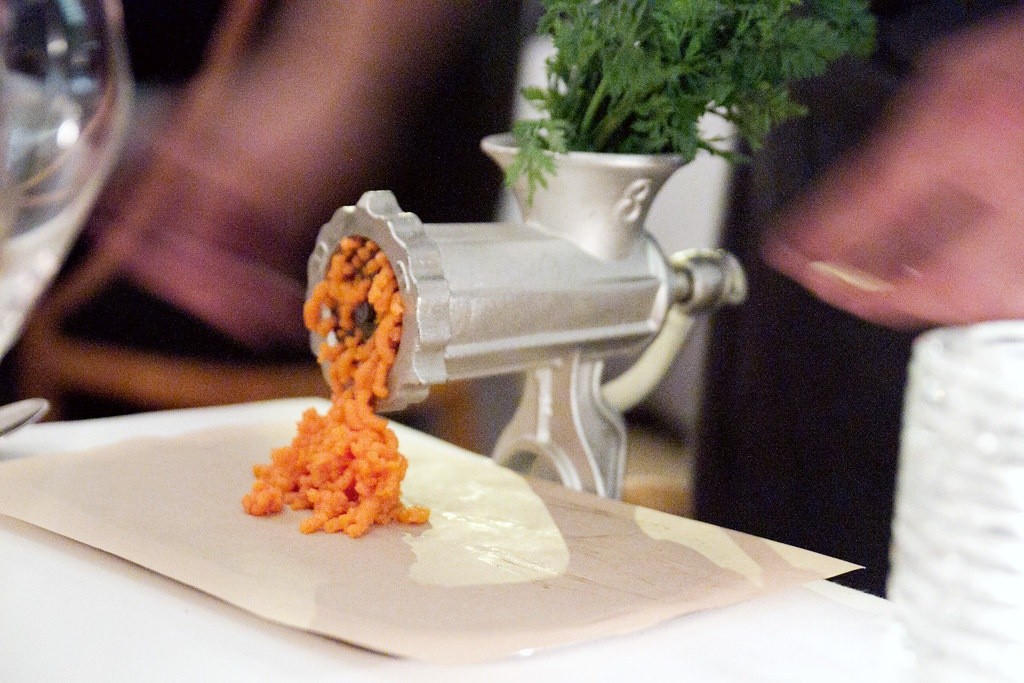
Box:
[500,0,878,211]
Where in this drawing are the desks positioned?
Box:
[0,393,894,683]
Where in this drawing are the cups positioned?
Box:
[1,1,128,363]
[886,319,1023,682]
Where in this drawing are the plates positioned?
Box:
[0,423,864,663]
